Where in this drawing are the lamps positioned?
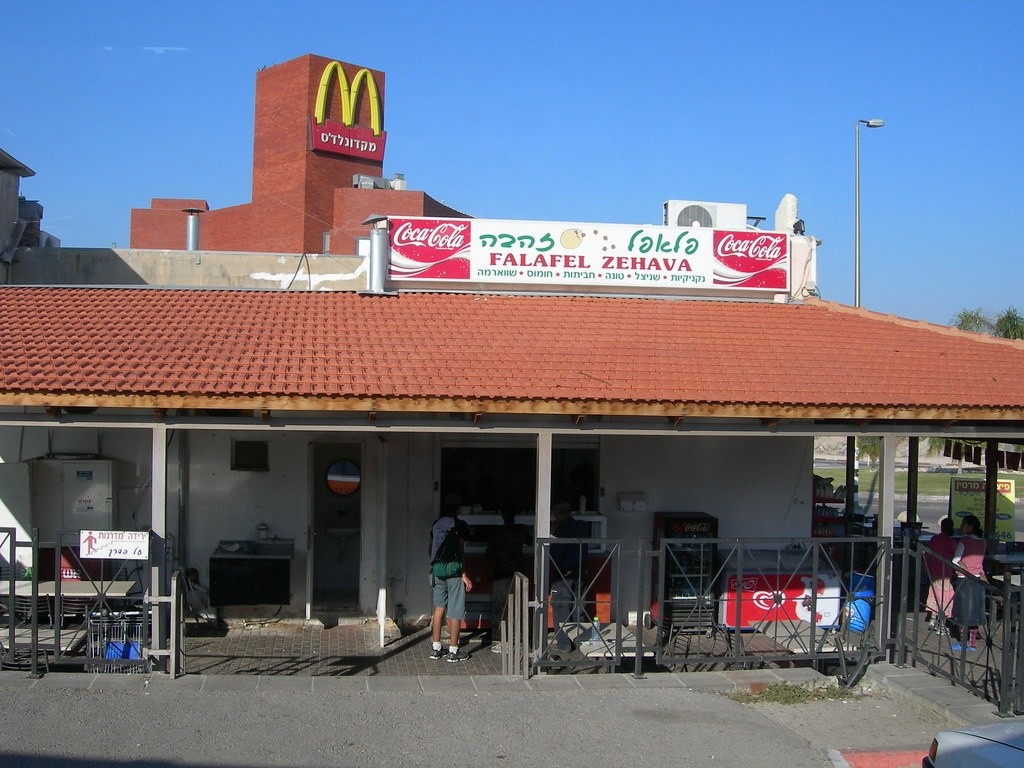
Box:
[793,219,806,235]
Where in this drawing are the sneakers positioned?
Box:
[446,649,470,662]
[429,646,449,660]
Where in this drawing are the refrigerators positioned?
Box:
[719,548,841,630]
[650,511,718,631]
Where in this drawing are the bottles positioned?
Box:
[823,544,836,562]
[816,524,833,537]
[591,617,600,640]
[580,495,586,514]
[815,505,839,518]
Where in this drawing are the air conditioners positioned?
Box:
[662,199,747,233]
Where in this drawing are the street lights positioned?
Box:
[851,118,888,513]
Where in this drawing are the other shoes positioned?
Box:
[520,642,531,651]
[948,642,968,651]
[548,644,571,653]
[967,646,977,651]
[491,641,514,654]
[928,619,940,631]
[935,626,954,636]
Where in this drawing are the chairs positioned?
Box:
[651,618,692,672]
[709,622,765,670]
[842,615,875,651]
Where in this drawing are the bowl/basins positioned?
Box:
[459,504,482,514]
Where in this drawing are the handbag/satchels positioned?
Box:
[432,519,465,577]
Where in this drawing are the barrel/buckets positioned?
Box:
[845,570,876,636]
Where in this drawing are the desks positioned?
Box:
[0,580,138,624]
[558,622,654,673]
[0,628,88,673]
[748,619,858,668]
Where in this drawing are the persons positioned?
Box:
[484,508,535,654]
[428,499,473,662]
[550,502,588,653]
[925,516,988,654]
